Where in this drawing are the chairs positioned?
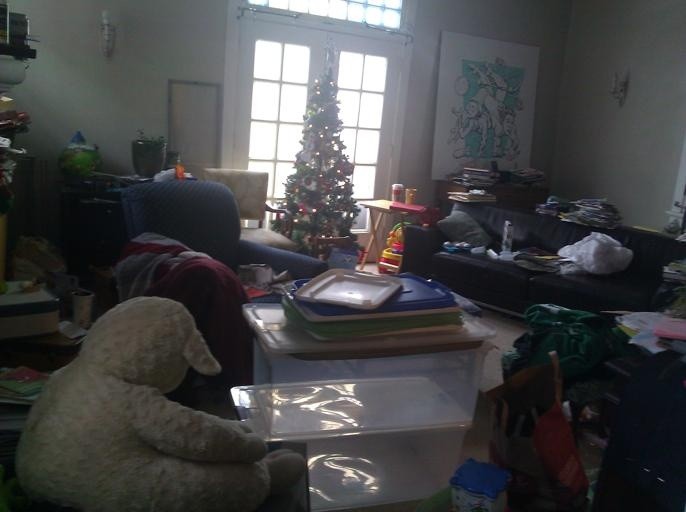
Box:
[205,168,298,252]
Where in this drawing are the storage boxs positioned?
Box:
[0,280,59,340]
[244,303,498,435]
[449,459,513,512]
[231,379,473,512]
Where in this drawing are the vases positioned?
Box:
[0,213,8,294]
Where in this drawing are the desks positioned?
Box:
[436,180,551,208]
[6,315,98,372]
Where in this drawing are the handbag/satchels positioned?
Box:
[537,391,590,512]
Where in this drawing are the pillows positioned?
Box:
[436,210,493,248]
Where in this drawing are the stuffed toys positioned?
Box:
[14,298,305,510]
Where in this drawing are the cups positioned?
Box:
[392,183,404,204]
[405,189,418,205]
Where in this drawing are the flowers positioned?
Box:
[0,96,32,205]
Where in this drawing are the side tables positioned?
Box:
[360,199,409,272]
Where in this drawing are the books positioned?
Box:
[447,189,497,204]
[0,364,49,406]
[513,165,544,188]
[453,166,500,188]
[563,196,622,231]
[616,311,686,355]
[662,259,685,283]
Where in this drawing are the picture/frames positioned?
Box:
[167,79,223,170]
[431,30,540,180]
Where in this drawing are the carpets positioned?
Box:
[355,262,531,390]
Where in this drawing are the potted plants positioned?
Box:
[131,129,165,177]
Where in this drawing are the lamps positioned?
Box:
[613,69,629,106]
[99,10,117,59]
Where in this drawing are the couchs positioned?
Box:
[121,181,328,394]
[404,202,686,321]
